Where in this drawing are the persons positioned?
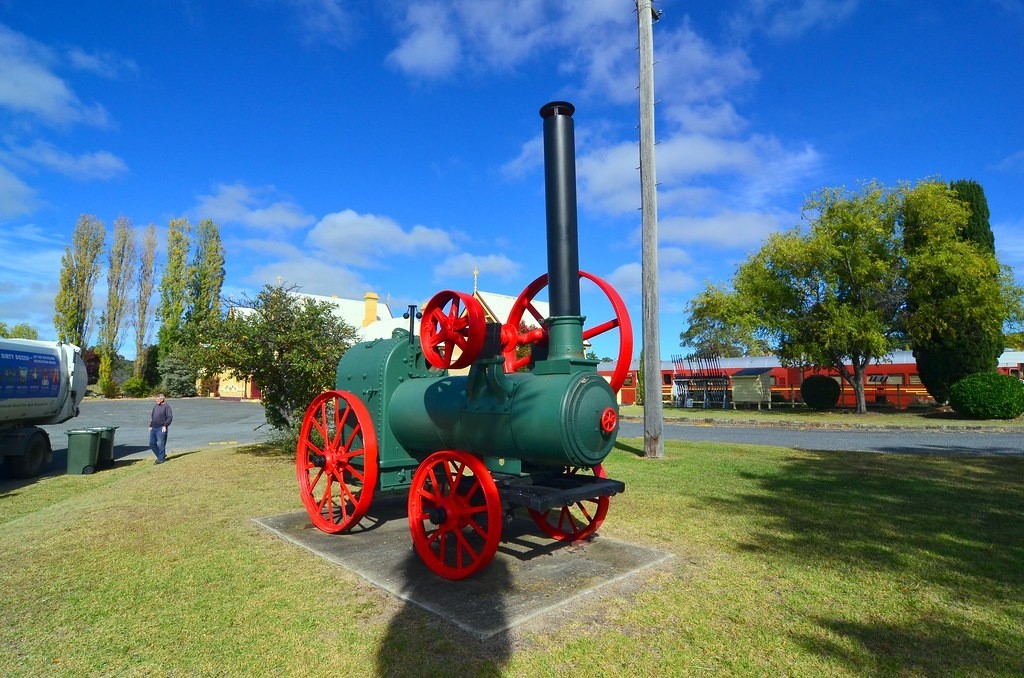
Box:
[148,394,173,466]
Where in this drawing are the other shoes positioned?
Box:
[154,459,163,465]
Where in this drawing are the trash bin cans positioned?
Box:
[86,426,120,469]
[64,429,104,475]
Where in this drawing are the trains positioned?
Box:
[295,102,633,580]
[597,347,1024,410]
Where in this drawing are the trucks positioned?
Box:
[0,337,87,479]
